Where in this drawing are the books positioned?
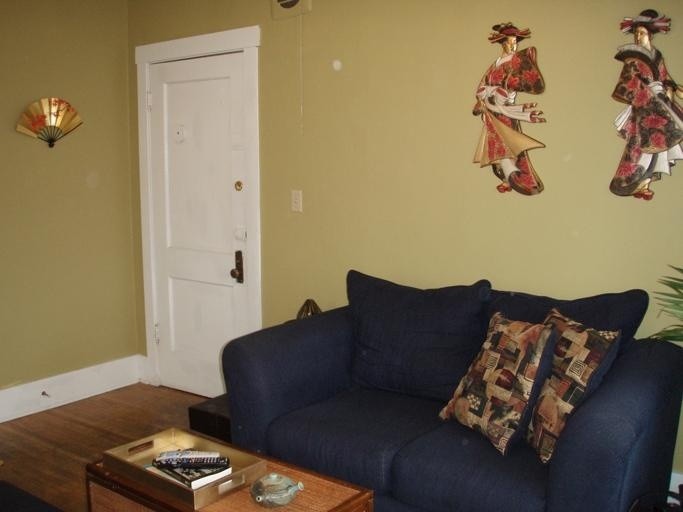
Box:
[152,447,231,487]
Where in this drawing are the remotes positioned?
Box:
[156,458,230,469]
[159,450,221,458]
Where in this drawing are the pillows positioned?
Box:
[492,288,647,355]
[439,311,555,455]
[347,271,492,402]
[526,307,620,464]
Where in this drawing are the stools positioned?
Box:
[189,394,230,442]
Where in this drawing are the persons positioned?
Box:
[609,9,682,201]
[472,22,544,196]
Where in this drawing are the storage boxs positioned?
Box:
[102,426,267,512]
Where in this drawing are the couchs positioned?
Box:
[222,306,683,511]
